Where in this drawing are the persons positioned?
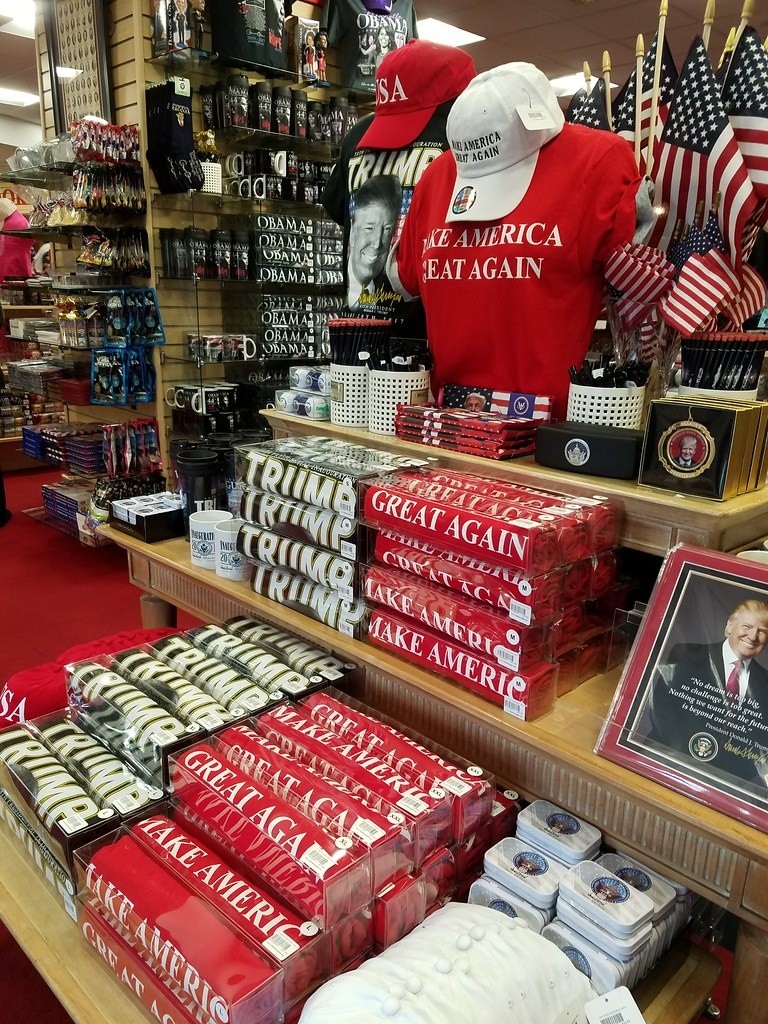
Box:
[314,30,328,82]
[359,29,406,76]
[265,0,286,37]
[345,174,404,313]
[638,598,767,813]
[672,435,698,467]
[463,394,486,411]
[227,339,242,360]
[191,0,207,51]
[0,198,36,293]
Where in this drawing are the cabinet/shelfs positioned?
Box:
[1,406,768,1024]
[0,0,375,541]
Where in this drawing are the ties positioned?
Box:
[726,660,744,719]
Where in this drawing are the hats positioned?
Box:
[444,62,565,223]
[356,37,477,149]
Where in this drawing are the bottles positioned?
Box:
[169,426,274,541]
[198,73,361,160]
[158,225,249,281]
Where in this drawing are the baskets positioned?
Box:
[566,382,645,430]
[368,367,430,435]
[330,363,370,427]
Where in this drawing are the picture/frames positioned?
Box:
[42,0,116,138]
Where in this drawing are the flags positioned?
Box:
[564,21,768,365]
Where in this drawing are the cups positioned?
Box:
[186,333,204,359]
[190,388,220,415]
[194,382,239,413]
[174,386,202,413]
[165,385,189,412]
[214,519,255,581]
[189,509,234,570]
[223,333,257,361]
[224,148,336,207]
[201,334,224,361]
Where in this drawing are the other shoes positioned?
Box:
[2,509,12,526]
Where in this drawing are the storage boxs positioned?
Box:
[0,216,768,1024]
